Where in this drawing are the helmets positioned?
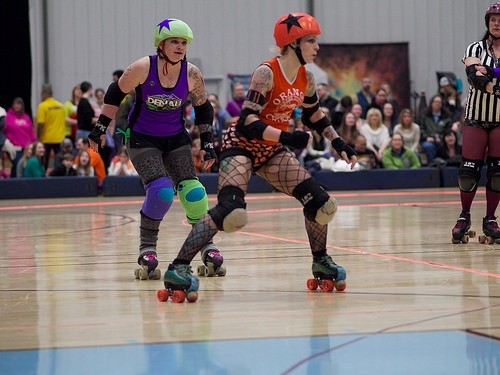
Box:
[274,12,321,48]
[152,18,194,48]
[484,2,500,29]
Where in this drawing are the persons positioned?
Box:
[451,5,500,240]
[0,69,140,185]
[293,73,466,174]
[86,19,224,269]
[164,12,361,292]
[185,81,246,175]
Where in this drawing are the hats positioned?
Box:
[439,76,457,87]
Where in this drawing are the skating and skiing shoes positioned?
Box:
[307,253,346,291]
[157,264,200,302]
[478,215,500,244]
[134,246,161,280]
[197,242,227,277]
[451,211,476,244]
